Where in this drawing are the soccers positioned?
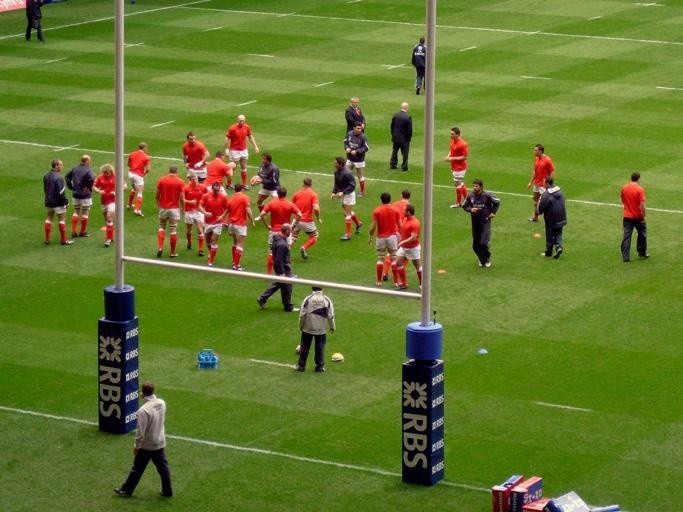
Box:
[332,352,343,363]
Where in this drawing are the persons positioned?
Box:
[537,176,567,260]
[330,96,369,240]
[527,144,554,222]
[126,114,323,311]
[25,0,46,42]
[444,127,469,208]
[461,179,500,268]
[294,286,336,373]
[412,38,426,95]
[390,102,412,172]
[113,382,172,497]
[42,154,116,247]
[369,188,422,293]
[620,172,650,262]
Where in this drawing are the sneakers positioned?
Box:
[115,487,127,496]
[300,246,307,259]
[376,275,406,290]
[553,248,562,259]
[639,253,649,258]
[529,217,538,222]
[340,221,362,240]
[45,233,113,246]
[127,204,143,216]
[157,246,245,270]
[227,184,249,190]
[450,204,460,208]
[257,297,264,310]
[479,260,491,267]
[292,307,299,312]
